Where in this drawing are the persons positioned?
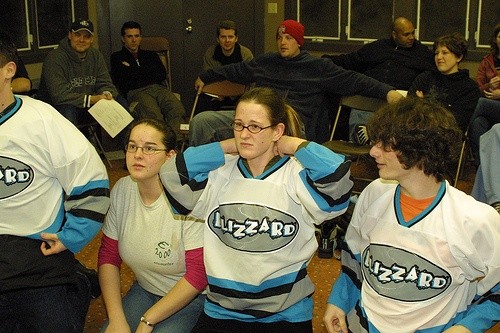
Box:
[0,27,110,333]
[197,21,254,113]
[322,97,500,333]
[320,16,432,143]
[355,32,481,145]
[470,123,500,216]
[11,55,32,92]
[189,19,406,147]
[109,20,187,134]
[41,18,129,171]
[159,86,353,333]
[471,25,500,170]
[98,119,207,333]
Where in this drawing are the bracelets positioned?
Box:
[141,316,157,328]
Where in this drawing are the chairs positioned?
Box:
[75,36,469,196]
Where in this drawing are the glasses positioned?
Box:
[125,143,169,155]
[231,121,278,134]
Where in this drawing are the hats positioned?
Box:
[71,19,95,37]
[276,21,304,46]
